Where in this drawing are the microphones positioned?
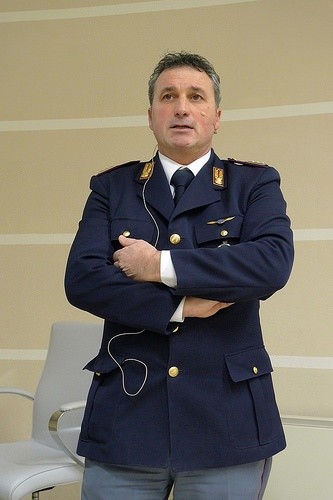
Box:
[200,111,217,132]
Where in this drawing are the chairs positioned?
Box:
[0,320,105,499]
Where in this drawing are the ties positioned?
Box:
[170,170,194,208]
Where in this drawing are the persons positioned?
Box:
[61,52,296,500]
